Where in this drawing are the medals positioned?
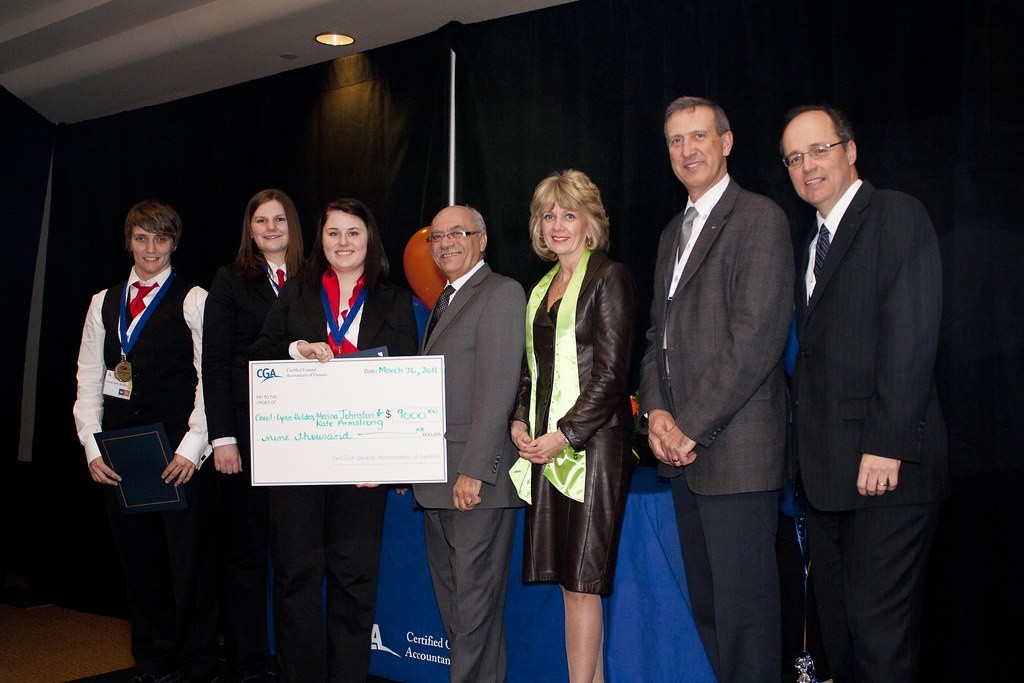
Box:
[114,361,132,382]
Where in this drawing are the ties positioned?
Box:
[129,282,159,320]
[678,206,699,262]
[276,269,285,288]
[814,224,830,278]
[424,285,455,346]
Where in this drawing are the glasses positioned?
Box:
[783,141,848,167]
[427,230,482,242]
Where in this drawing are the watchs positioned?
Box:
[563,434,571,444]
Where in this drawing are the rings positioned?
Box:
[467,502,472,505]
[672,458,679,462]
[321,348,327,352]
[877,482,888,486]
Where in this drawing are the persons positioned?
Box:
[640,96,793,683]
[73,202,215,683]
[781,105,944,683]
[396,206,527,683]
[508,170,627,683]
[274,201,418,682]
[204,189,328,683]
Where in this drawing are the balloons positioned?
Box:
[403,226,448,309]
